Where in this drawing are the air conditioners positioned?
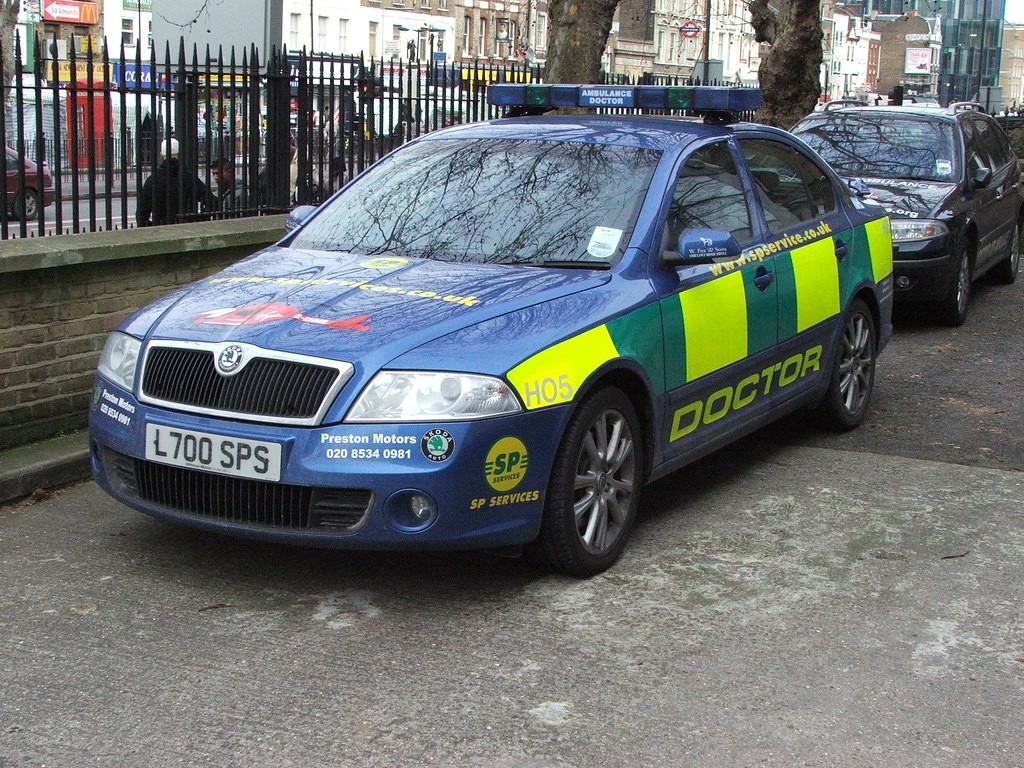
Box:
[41,39,67,60]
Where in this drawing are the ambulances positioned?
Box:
[86,82,893,579]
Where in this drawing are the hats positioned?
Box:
[161,138,178,155]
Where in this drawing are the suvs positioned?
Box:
[753,99,1024,328]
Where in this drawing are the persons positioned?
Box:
[401,98,415,135]
[210,156,249,216]
[323,156,350,196]
[310,105,332,129]
[135,137,220,227]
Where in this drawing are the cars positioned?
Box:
[991,116,1024,184]
[6,147,56,221]
